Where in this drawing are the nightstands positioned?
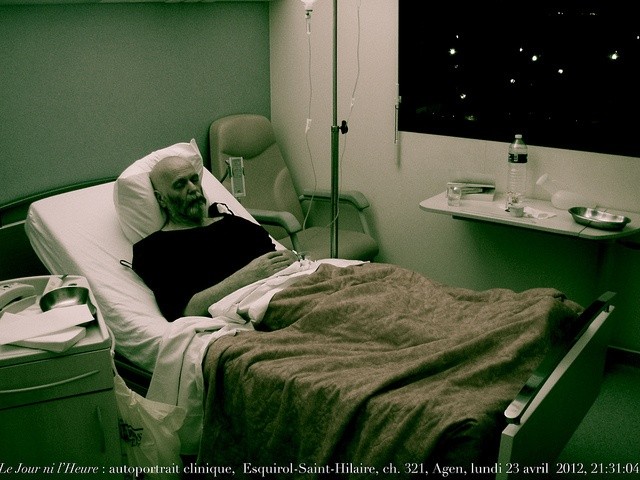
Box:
[1,274,124,479]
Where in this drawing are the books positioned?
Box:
[447,182,496,201]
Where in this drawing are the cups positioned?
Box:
[447,184,461,207]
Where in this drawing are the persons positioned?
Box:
[131,155,301,322]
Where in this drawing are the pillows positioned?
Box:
[112,138,203,244]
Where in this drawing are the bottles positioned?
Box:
[507,134,528,204]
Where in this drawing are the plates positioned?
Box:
[39,287,97,328]
[568,206,631,230]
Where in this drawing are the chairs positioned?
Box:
[208,113,380,266]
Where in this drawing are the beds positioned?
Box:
[0,175,616,480]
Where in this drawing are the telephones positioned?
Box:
[0,282,37,321]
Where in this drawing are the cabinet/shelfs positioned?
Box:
[418,187,640,241]
